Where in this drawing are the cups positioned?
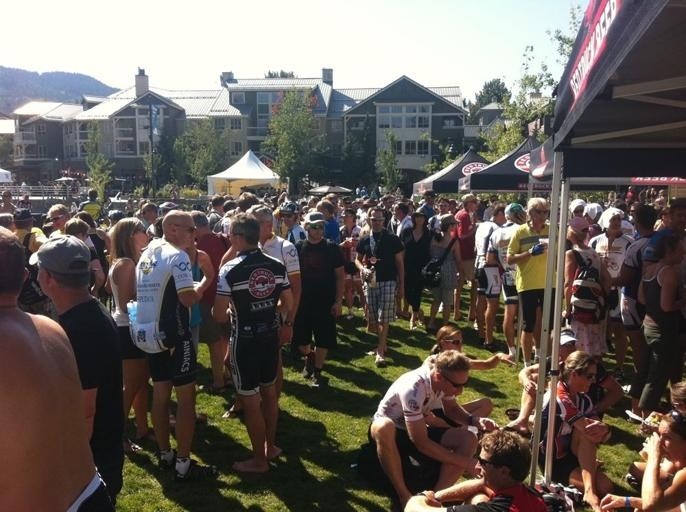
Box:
[352,238,358,247]
[538,238,549,253]
[345,237,352,247]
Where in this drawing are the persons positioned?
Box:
[2,192,686,511]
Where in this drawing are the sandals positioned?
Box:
[198,384,226,395]
[374,350,386,368]
[482,341,500,353]
[209,375,233,387]
[366,345,390,357]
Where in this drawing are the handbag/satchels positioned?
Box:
[420,256,444,291]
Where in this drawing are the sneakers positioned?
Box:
[303,352,314,379]
[159,447,177,470]
[310,367,321,392]
[173,457,201,486]
[615,368,625,382]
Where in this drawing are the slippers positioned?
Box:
[498,422,532,440]
[505,408,535,421]
[222,409,242,420]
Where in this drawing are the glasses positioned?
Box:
[49,214,64,222]
[476,456,494,467]
[230,231,242,237]
[584,372,598,382]
[532,206,550,215]
[278,200,448,230]
[574,208,584,214]
[444,376,468,388]
[444,338,464,346]
[168,223,196,233]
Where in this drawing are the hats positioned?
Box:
[27,235,92,275]
[442,214,462,224]
[568,215,590,235]
[461,193,481,203]
[13,208,32,222]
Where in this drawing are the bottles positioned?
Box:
[639,437,662,462]
[126,299,137,329]
[368,266,379,288]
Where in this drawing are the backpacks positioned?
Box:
[569,248,607,327]
